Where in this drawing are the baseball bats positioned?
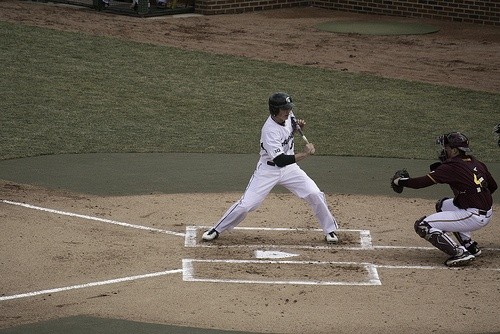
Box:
[290,111,316,156]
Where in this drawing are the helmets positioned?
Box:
[436,132,469,152]
[269,92,296,116]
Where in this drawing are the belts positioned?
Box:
[267,161,275,166]
[464,208,487,215]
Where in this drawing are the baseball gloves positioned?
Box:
[390,168,409,194]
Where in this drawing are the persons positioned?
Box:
[202,92,339,243]
[391,132,498,267]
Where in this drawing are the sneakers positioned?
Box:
[325,232,338,243]
[202,228,218,240]
[446,251,476,267]
[467,246,482,257]
